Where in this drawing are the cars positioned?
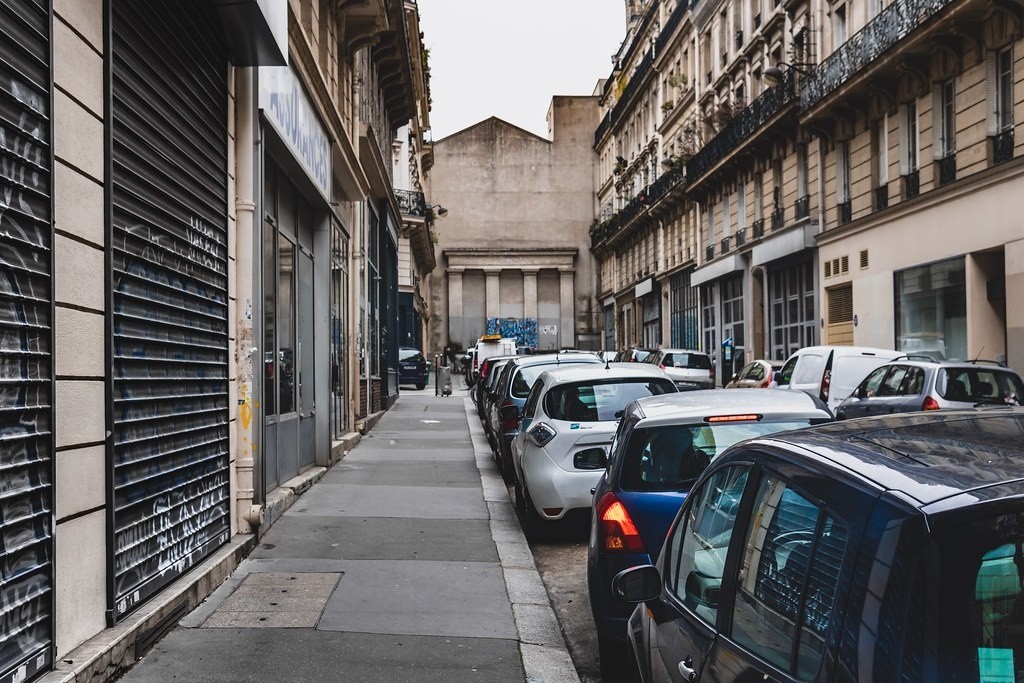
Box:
[584,386,840,683]
[399,346,428,391]
[610,400,1024,683]
[834,353,1024,424]
[450,335,715,483]
[724,359,790,389]
[498,360,686,530]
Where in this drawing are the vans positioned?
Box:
[766,345,909,418]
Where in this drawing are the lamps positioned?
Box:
[426,204,448,217]
[661,154,686,172]
